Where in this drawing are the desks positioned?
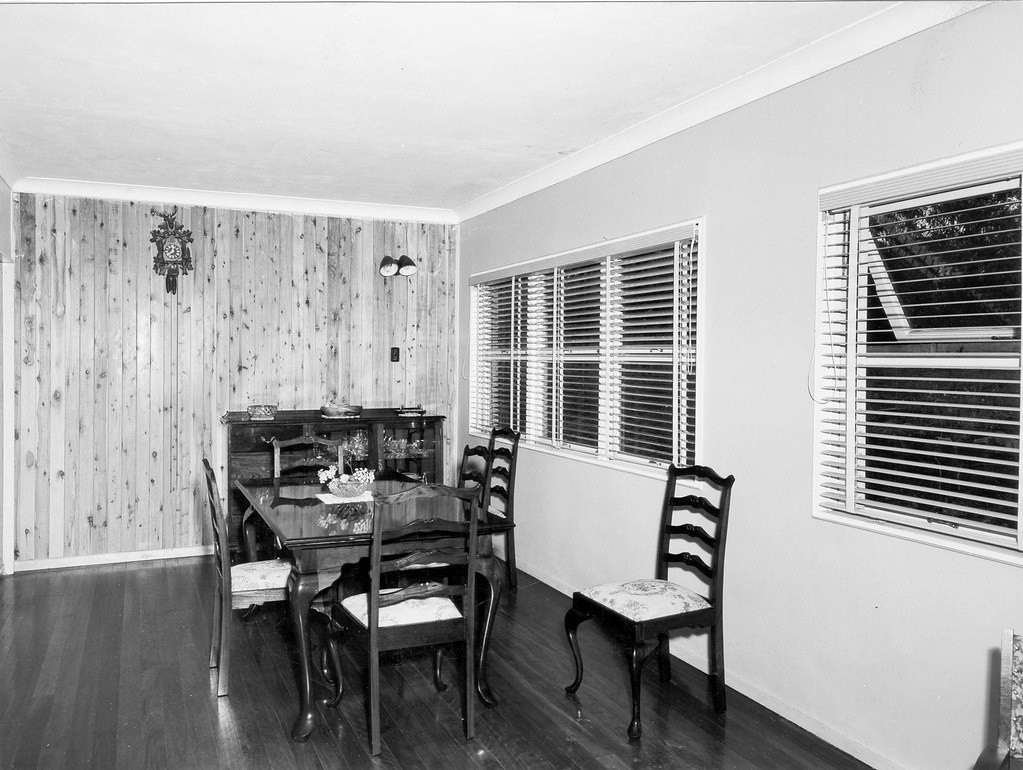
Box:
[233,465,517,744]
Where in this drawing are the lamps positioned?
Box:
[378,255,418,277]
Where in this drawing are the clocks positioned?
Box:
[150,206,195,296]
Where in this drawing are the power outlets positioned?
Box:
[390,346,399,363]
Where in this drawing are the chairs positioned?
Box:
[321,495,479,758]
[974,629,1023,770]
[201,457,335,696]
[564,462,735,740]
[458,444,494,512]
[259,433,347,628]
[469,426,521,582]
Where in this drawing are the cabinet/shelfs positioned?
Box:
[221,411,447,560]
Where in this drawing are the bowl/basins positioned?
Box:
[247,405,278,417]
[320,405,363,415]
